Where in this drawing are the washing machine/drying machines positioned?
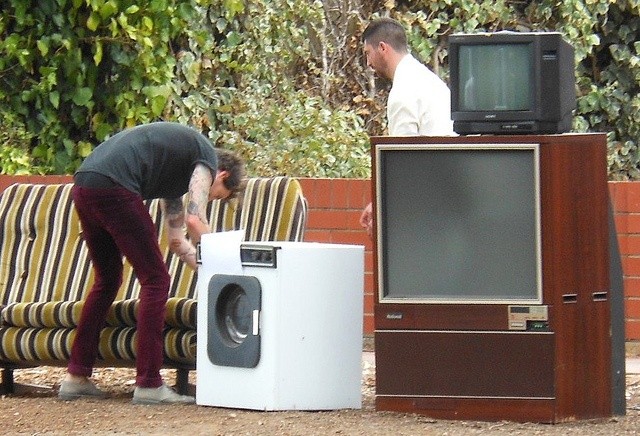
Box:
[195,243,365,415]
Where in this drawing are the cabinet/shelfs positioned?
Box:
[369,130,625,424]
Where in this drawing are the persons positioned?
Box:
[58,121,240,403]
[360,18,481,225]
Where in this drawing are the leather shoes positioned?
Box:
[58,379,110,401]
[132,386,196,405]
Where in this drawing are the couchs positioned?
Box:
[1,176,309,392]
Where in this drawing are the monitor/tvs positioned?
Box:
[370,134,613,331]
[449,34,576,134]
